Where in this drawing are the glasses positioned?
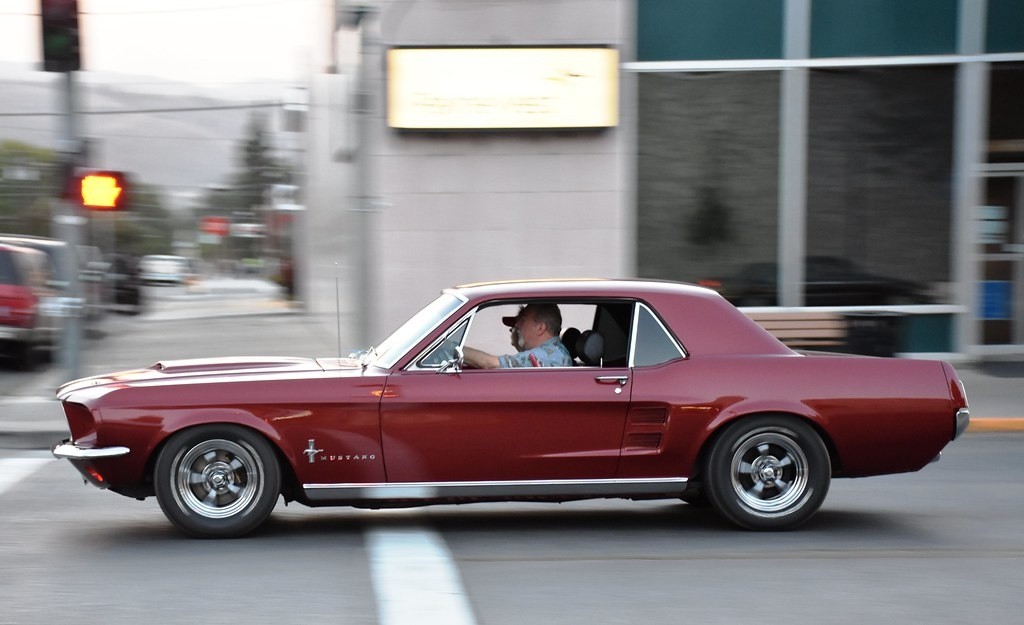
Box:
[509,329,518,333]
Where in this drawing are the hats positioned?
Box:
[502,313,520,328]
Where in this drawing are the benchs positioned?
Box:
[742,312,849,354]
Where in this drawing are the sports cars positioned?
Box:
[50,280,972,540]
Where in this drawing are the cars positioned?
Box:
[1,233,139,369]
[701,254,946,307]
[137,254,191,286]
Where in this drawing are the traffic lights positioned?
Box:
[42,1,82,72]
[78,169,124,210]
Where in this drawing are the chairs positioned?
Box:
[560,328,607,369]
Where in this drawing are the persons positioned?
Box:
[439,303,573,368]
